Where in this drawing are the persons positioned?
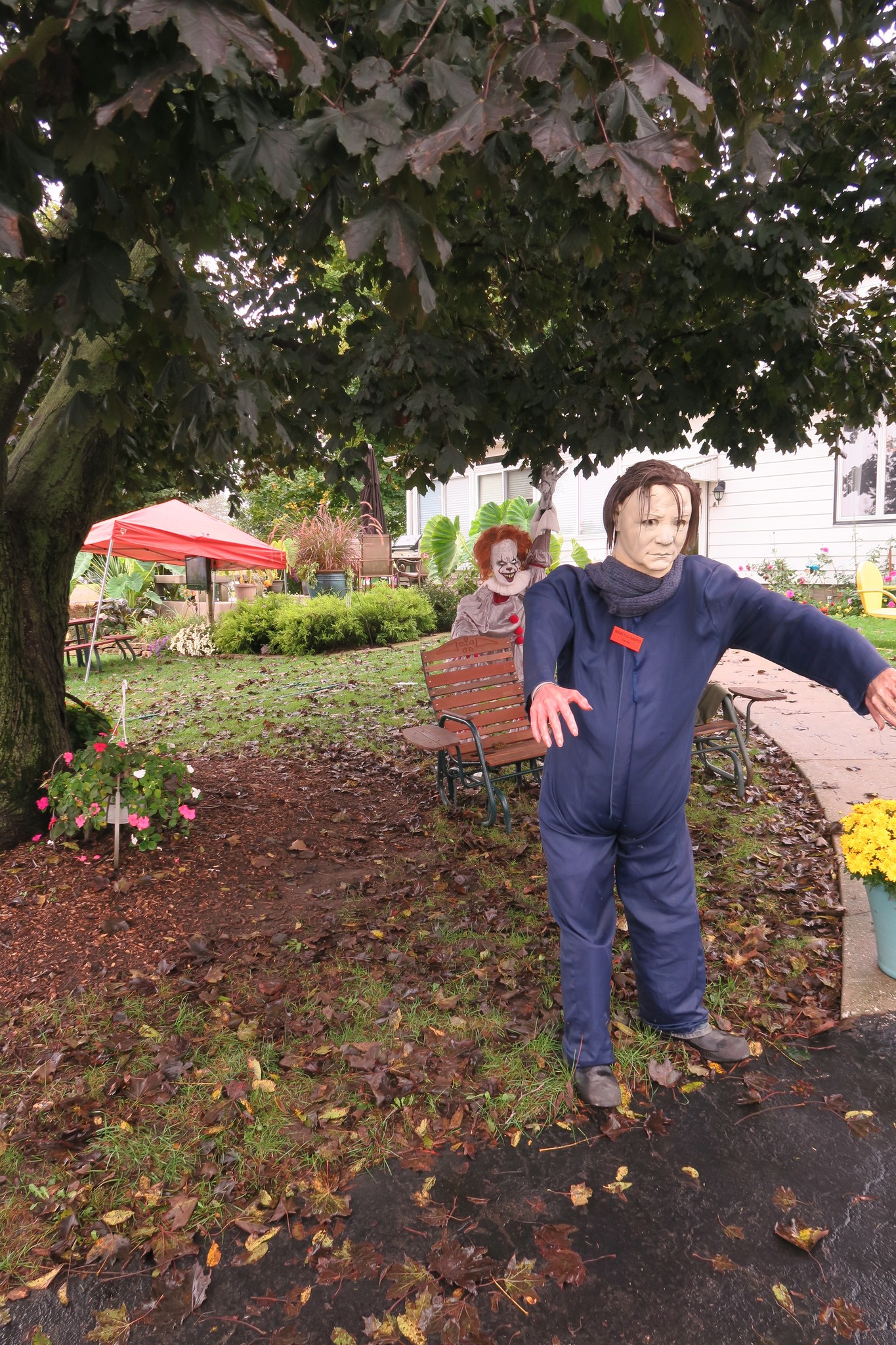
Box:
[444,525,548,778]
[525,460,896,1108]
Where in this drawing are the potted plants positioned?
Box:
[233,570,259,603]
[260,491,384,596]
[265,539,285,594]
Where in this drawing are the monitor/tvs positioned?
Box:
[185,554,212,591]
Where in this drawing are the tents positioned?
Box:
[79,499,287,683]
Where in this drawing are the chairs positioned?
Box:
[396,550,431,590]
[355,533,395,592]
[406,635,548,826]
[693,687,785,795]
[856,562,896,620]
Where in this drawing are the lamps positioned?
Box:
[712,478,729,507]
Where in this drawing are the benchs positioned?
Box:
[101,633,143,662]
[63,636,90,666]
[63,639,116,674]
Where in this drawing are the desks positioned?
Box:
[153,573,232,603]
[63,616,103,668]
